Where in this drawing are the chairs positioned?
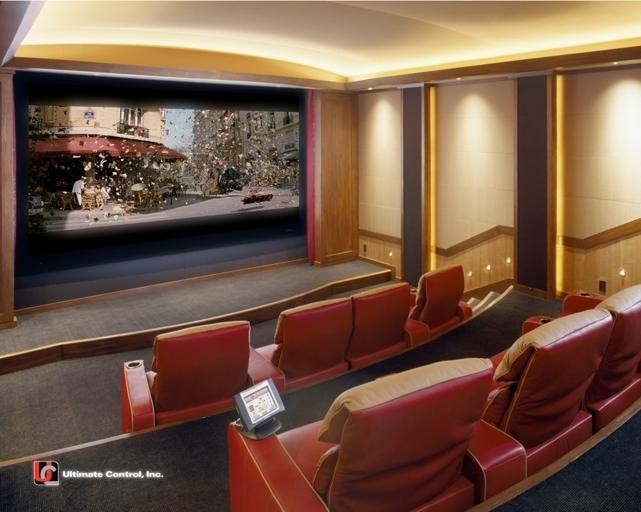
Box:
[121,264,641,512]
